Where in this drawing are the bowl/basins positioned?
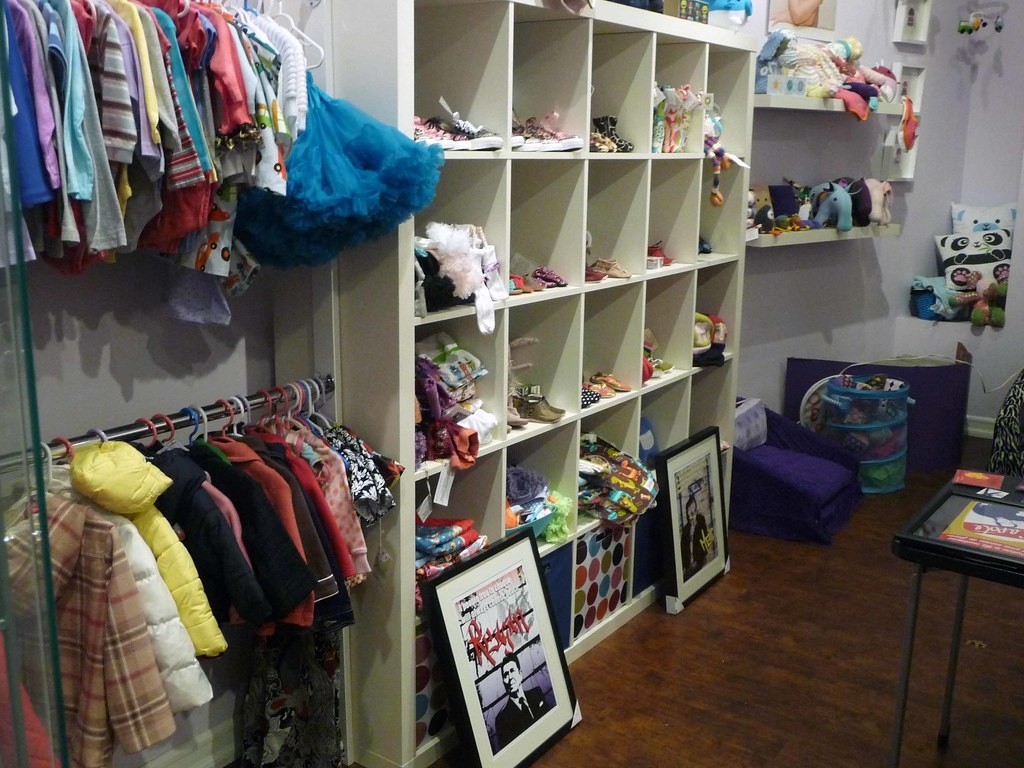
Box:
[506,503,557,540]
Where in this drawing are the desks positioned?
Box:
[887,468,1024,768]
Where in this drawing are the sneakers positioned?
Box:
[512,109,562,151]
[432,97,503,150]
[413,115,471,150]
[540,110,584,150]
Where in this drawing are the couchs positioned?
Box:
[729,397,865,547]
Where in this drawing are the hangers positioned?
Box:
[177,0,325,71]
[38,440,54,466]
[269,3,761,768]
[180,377,337,445]
[87,428,107,448]
[51,437,72,458]
[136,413,176,448]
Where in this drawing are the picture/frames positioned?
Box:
[891,0,932,47]
[418,526,583,768]
[766,0,838,43]
[881,126,921,182]
[889,62,929,117]
[655,426,731,615]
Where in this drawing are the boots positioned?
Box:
[593,114,634,154]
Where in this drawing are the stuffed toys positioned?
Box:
[704,116,730,206]
[754,29,898,120]
[949,273,1007,328]
[747,175,893,237]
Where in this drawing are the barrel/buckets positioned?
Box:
[823,376,911,494]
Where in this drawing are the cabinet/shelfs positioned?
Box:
[747,94,902,250]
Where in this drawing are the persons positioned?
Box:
[493,653,549,750]
[973,501,1024,529]
[681,500,709,582]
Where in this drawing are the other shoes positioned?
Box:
[507,404,528,434]
[650,241,676,266]
[514,384,566,424]
[590,372,631,393]
[510,266,568,294]
[585,268,606,281]
[587,258,632,279]
[591,130,617,154]
[699,237,713,254]
[642,346,675,374]
[581,388,601,409]
[582,379,616,399]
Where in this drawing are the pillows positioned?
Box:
[934,225,1013,290]
[951,202,1016,235]
